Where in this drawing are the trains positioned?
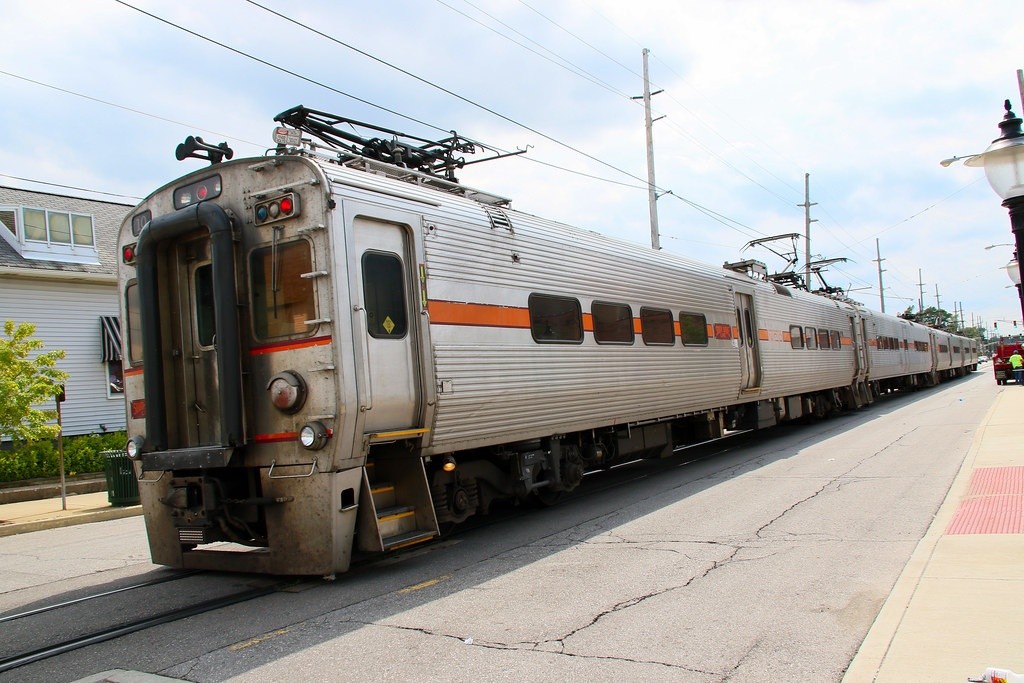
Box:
[116,105,978,580]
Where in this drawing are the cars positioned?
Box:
[978,355,989,364]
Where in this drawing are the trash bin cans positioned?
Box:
[100,450,141,507]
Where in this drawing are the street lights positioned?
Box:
[964,97,1024,319]
[1006,249,1024,316]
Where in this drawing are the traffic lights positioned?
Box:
[993,322,997,330]
[1013,321,1016,328]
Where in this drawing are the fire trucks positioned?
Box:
[991,334,1024,385]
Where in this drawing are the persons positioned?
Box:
[1009,350,1023,385]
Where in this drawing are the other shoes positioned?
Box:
[1015,381,1023,384]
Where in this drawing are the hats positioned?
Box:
[1013,350,1018,354]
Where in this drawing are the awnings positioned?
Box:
[100,316,122,361]
